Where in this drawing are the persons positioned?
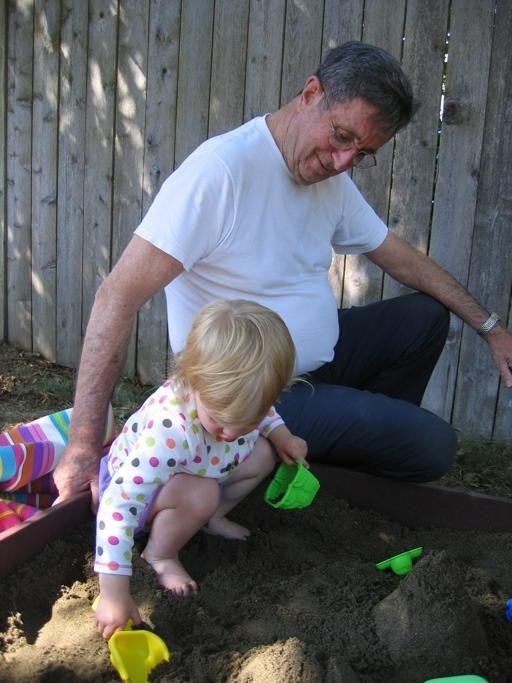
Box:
[92,298,309,644]
[46,43,512,509]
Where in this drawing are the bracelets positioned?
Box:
[476,309,502,338]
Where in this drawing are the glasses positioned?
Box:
[322,89,378,170]
